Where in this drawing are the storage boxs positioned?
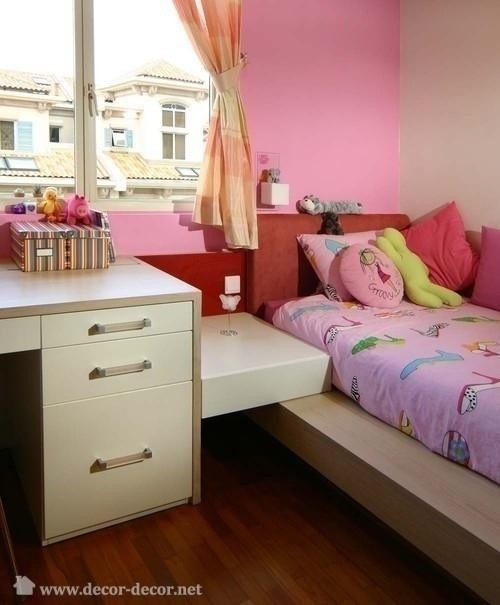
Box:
[7,219,111,271]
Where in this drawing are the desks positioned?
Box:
[0,256,202,547]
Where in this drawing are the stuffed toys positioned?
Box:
[296,194,365,215]
[376,227,463,309]
[65,193,91,226]
[317,210,345,236]
[37,188,63,223]
[266,166,282,184]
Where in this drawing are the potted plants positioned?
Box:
[14,187,26,198]
[32,183,43,197]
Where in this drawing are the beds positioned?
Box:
[248,212,500,605]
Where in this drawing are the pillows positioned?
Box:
[296,202,500,311]
